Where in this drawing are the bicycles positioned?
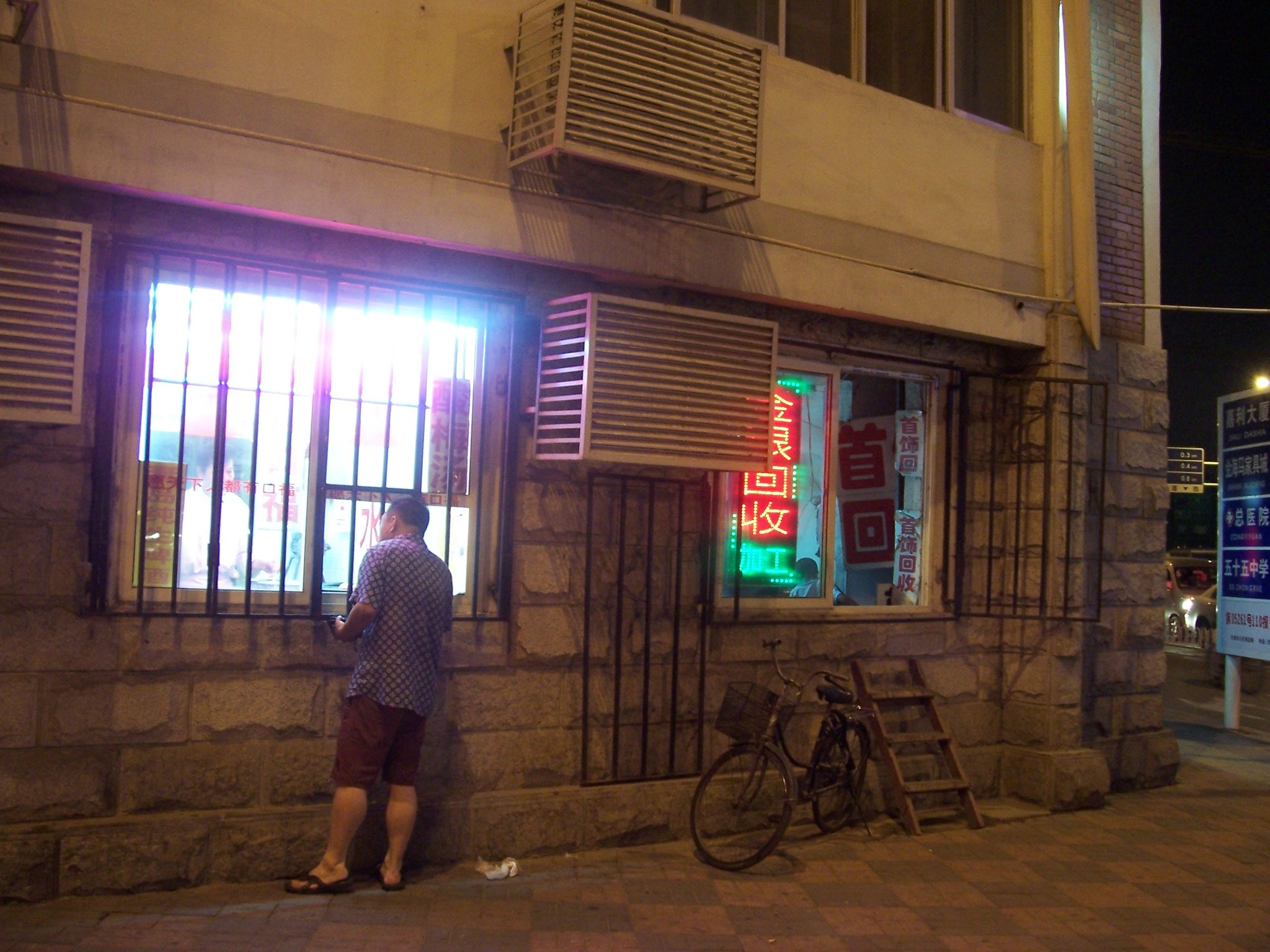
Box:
[686,637,883,871]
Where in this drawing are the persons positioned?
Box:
[788,557,859,607]
[284,498,453,895]
[1165,567,1190,589]
[177,444,278,592]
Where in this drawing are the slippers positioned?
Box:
[372,858,406,892]
[283,870,354,892]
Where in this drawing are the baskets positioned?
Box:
[714,679,794,741]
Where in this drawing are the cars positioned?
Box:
[1165,558,1217,645]
[1184,584,1217,649]
